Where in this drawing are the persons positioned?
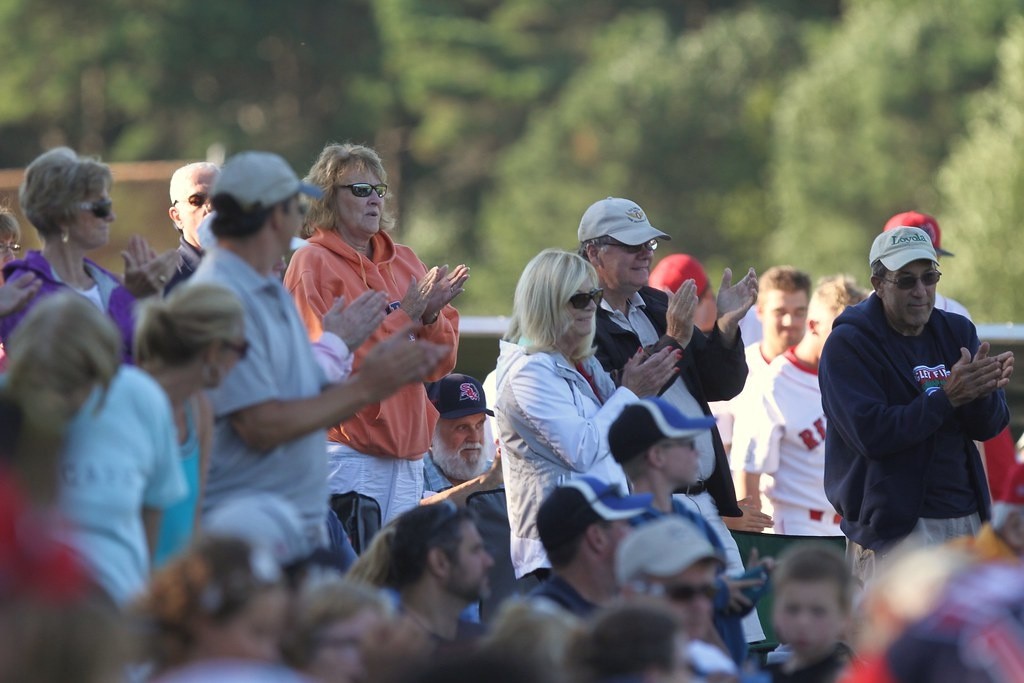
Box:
[0,141,1023,683]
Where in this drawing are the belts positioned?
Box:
[672,481,707,494]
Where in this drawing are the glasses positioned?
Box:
[222,341,249,359]
[585,484,619,508]
[75,199,112,217]
[173,192,210,206]
[569,289,604,309]
[0,243,23,255]
[583,238,658,253]
[873,269,942,289]
[338,183,387,198]
[643,442,694,459]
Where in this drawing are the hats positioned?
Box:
[424,373,494,419]
[577,196,672,245]
[647,254,707,298]
[883,211,954,257]
[209,494,346,586]
[869,226,940,271]
[608,398,716,463]
[536,477,652,552]
[211,152,324,212]
